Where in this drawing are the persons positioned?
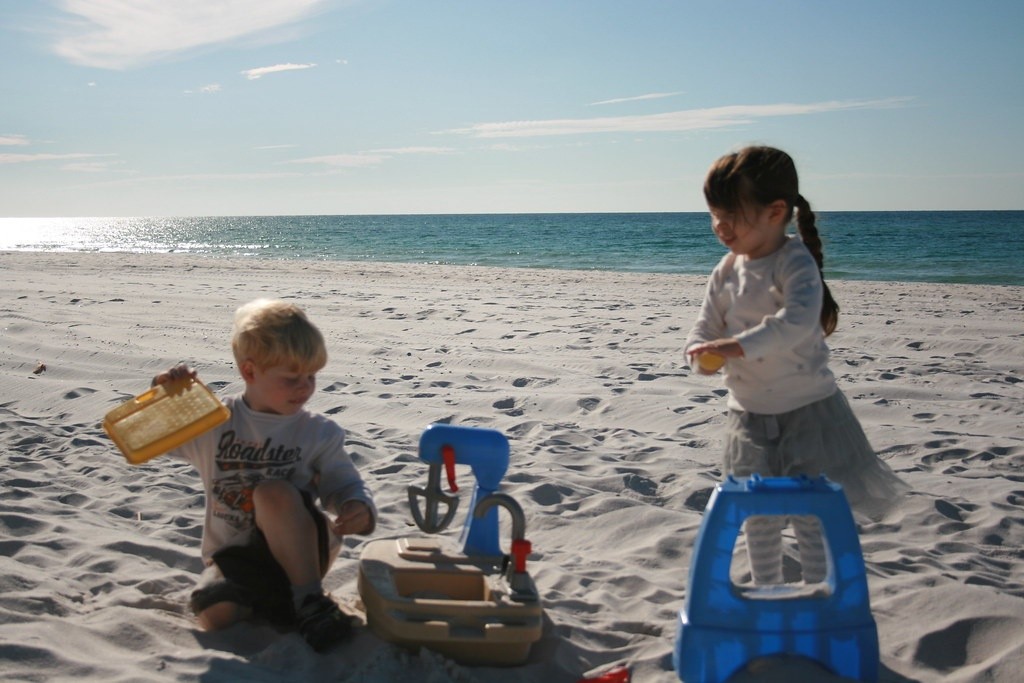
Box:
[683,146,913,588]
[151,302,378,652]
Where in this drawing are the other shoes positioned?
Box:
[290,589,363,654]
[258,583,296,634]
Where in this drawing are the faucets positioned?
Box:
[474,493,537,601]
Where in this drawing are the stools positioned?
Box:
[672,474,880,683]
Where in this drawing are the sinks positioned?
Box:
[389,567,496,601]
